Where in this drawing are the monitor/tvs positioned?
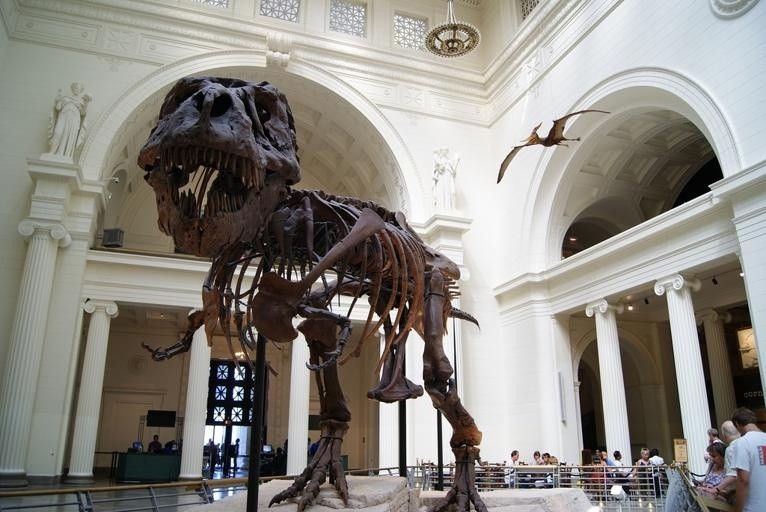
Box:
[262,444,272,452]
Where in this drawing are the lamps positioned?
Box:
[424,0,482,58]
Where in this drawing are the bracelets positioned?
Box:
[715,487,719,493]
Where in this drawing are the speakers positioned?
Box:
[146,410,176,427]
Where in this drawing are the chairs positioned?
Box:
[414,455,688,503]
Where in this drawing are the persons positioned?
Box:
[703,428,725,463]
[731,404,766,512]
[308,438,312,455]
[147,435,161,452]
[206,437,240,474]
[431,144,460,213]
[695,420,741,506]
[502,445,664,502]
[282,439,287,456]
[45,79,95,162]
[266,447,283,476]
[689,441,726,489]
